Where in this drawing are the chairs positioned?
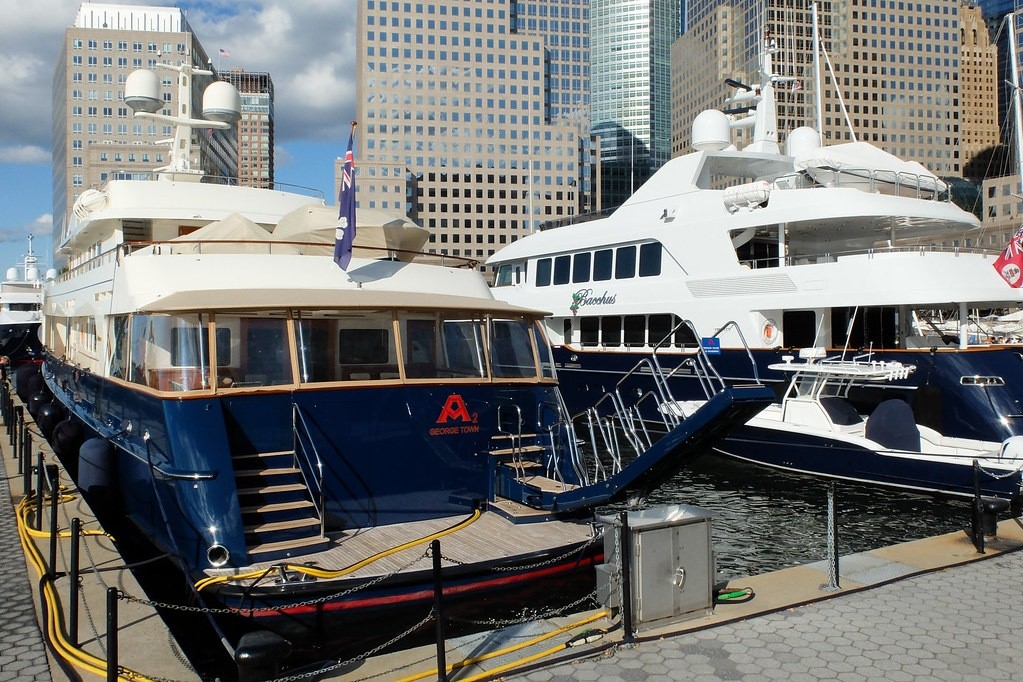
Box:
[245,374,268,385]
[349,371,371,379]
[379,371,400,379]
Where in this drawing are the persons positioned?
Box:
[985,337,1022,344]
[0,356,10,415]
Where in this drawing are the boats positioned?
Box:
[487,3,1023,520]
[18,37,770,678]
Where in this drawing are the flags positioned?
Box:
[992,224,1023,288]
[334,121,356,270]
